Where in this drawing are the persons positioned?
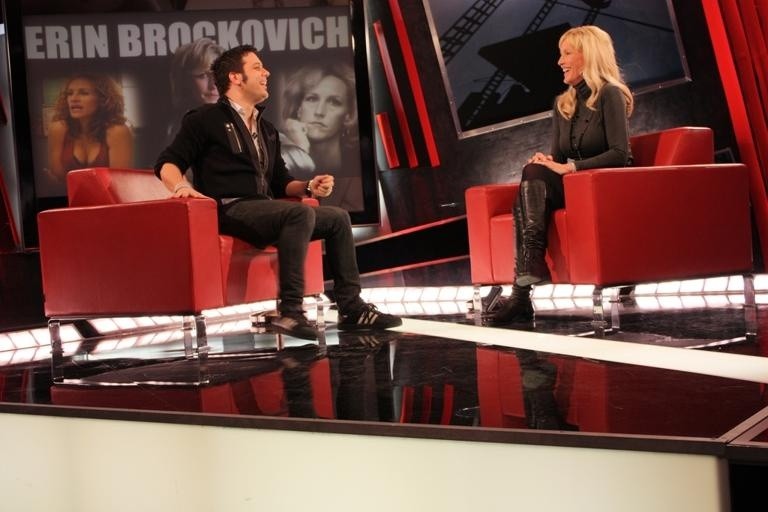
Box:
[47,72,135,196]
[164,39,225,106]
[282,56,363,209]
[154,47,402,339]
[486,25,631,325]
[271,329,402,422]
[478,340,575,431]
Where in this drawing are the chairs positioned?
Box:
[476,335,761,435]
[50,328,336,418]
[465,122,756,332]
[34,168,326,362]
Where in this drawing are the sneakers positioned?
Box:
[338,331,400,351]
[338,304,402,331]
[277,345,327,368]
[269,311,318,342]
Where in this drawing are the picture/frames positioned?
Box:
[0,0,381,248]
[420,0,698,141]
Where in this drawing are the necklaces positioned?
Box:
[570,104,595,160]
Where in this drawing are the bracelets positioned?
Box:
[303,179,316,195]
[173,184,194,191]
[565,156,576,172]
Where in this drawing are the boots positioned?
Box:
[488,180,550,323]
[515,349,577,429]
